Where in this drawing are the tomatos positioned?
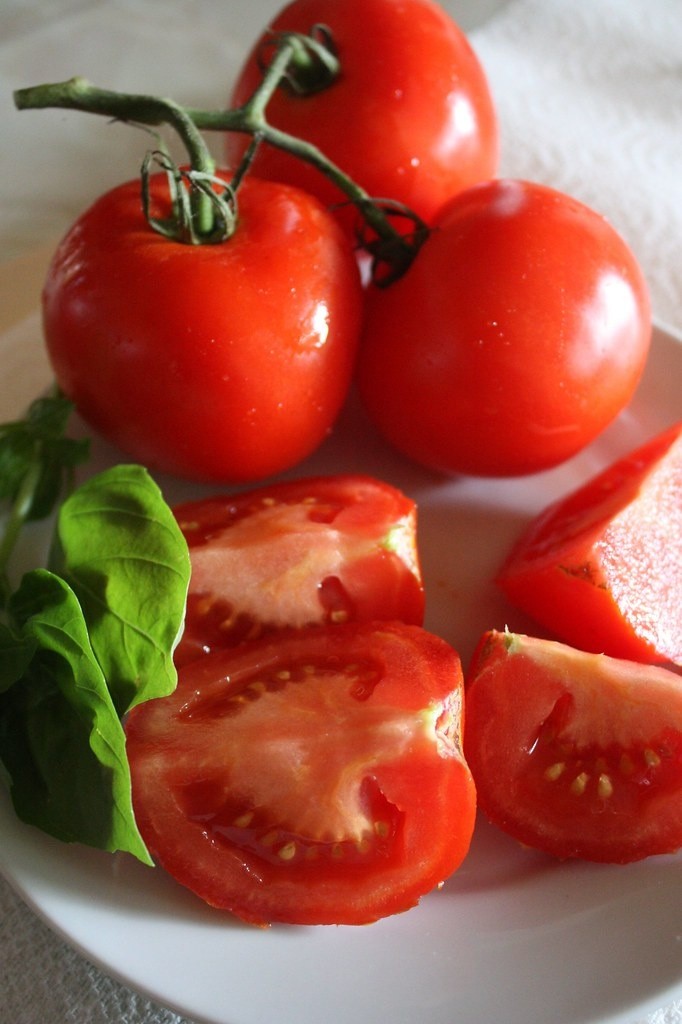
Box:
[10,1,682,925]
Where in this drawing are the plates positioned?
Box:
[0,303,682,1023]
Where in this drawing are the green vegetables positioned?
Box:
[2,383,194,870]
